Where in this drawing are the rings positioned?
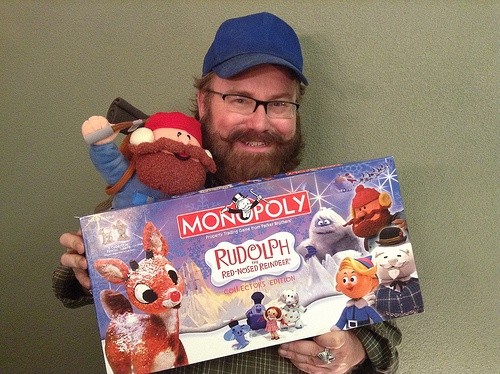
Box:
[317,347,335,365]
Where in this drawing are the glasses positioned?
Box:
[206,88,300,119]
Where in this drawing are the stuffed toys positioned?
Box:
[81,112,216,211]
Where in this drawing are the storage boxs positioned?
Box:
[78,156,425,374]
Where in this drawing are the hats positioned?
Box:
[202,12,308,86]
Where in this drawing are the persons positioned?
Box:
[51,11,402,374]
